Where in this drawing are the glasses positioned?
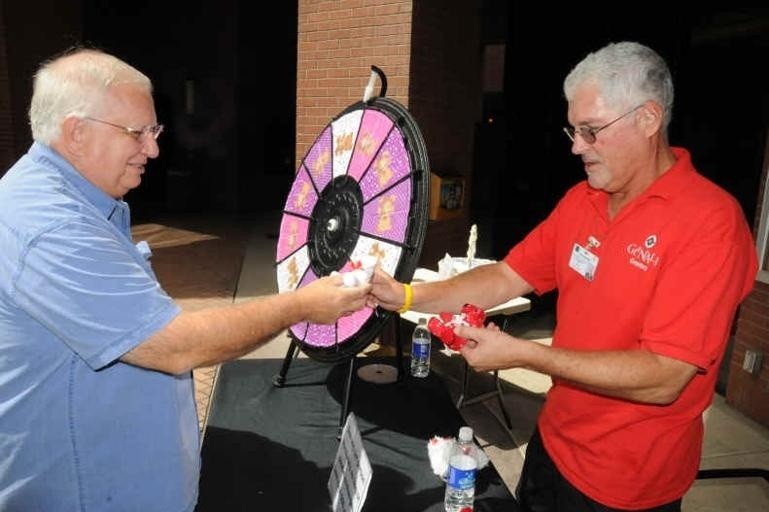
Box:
[87,117,164,141]
[563,104,644,144]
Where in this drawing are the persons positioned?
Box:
[1,39,371,511]
[368,39,760,512]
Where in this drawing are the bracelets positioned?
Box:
[396,283,412,315]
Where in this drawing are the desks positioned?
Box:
[394,280,532,430]
[193,356,520,511]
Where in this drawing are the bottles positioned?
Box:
[410,318,432,380]
[443,425,479,511]
